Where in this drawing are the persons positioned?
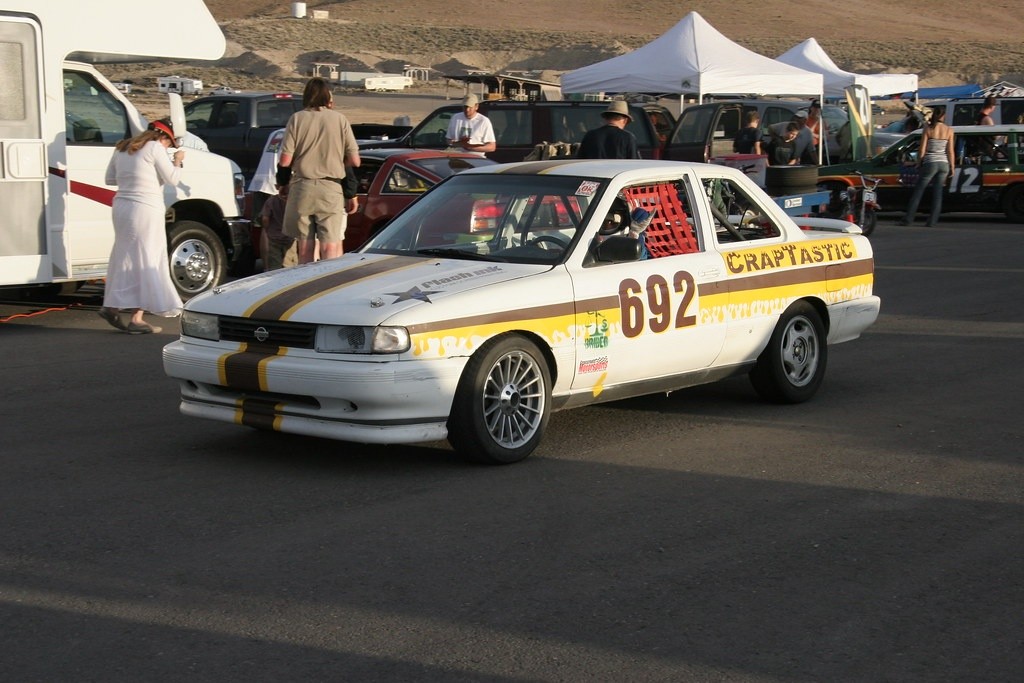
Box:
[579,100,641,160]
[247,129,306,272]
[445,93,496,157]
[1014,112,1024,154]
[894,105,955,228]
[279,77,360,272]
[733,111,763,154]
[649,115,668,160]
[903,118,919,135]
[765,122,799,166]
[806,100,819,166]
[974,97,1001,157]
[768,110,819,166]
[99,118,184,336]
[595,197,657,265]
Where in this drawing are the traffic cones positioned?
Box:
[799,213,811,230]
[847,215,853,222]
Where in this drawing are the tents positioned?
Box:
[561,10,824,171]
[773,34,985,162]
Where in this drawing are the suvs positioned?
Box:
[880,97,1024,135]
[357,100,745,184]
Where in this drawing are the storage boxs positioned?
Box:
[707,154,770,188]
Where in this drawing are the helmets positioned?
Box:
[596,195,630,240]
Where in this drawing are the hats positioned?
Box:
[462,93,479,107]
[984,97,999,106]
[601,101,633,122]
[151,119,179,148]
[813,100,821,108]
[794,110,809,119]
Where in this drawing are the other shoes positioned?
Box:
[127,321,162,335]
[98,306,128,331]
[896,220,912,226]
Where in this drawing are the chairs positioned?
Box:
[260,110,272,122]
[389,169,425,192]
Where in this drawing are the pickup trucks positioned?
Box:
[185,91,414,195]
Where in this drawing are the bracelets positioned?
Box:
[174,156,182,163]
[350,196,358,201]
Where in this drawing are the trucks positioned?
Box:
[0,0,252,305]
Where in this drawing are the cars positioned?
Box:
[209,86,234,96]
[343,149,582,254]
[811,124,1024,223]
[161,159,882,469]
[740,101,918,164]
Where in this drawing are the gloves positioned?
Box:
[629,207,655,240]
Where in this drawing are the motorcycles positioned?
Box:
[703,164,758,215]
[826,166,888,238]
